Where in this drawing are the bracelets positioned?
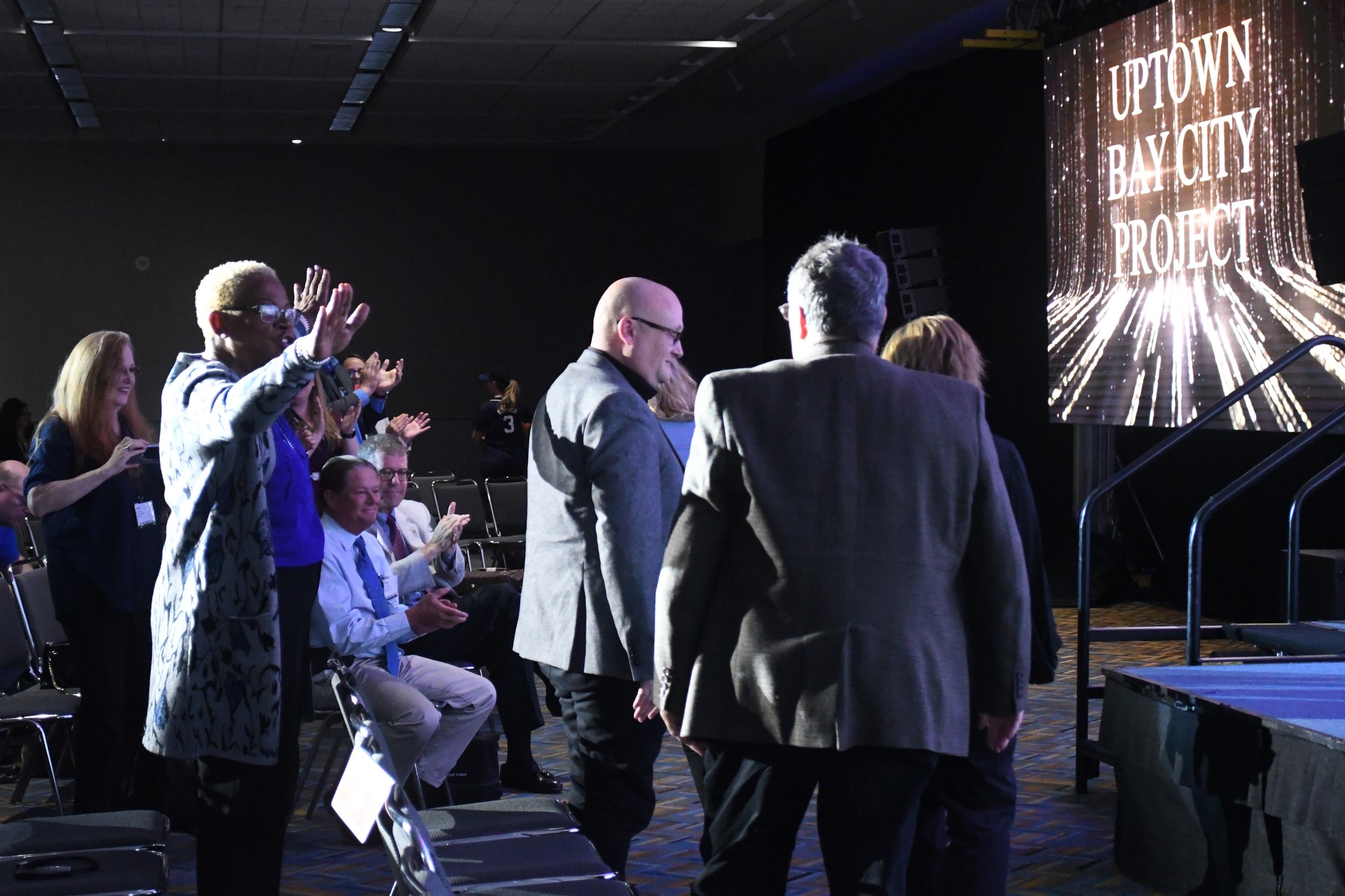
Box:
[295,338,331,369]
[373,391,388,399]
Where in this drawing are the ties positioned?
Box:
[353,535,400,677]
[386,515,407,561]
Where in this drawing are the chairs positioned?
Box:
[0,464,637,896]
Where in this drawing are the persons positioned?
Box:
[138,258,371,896]
[24,329,171,817]
[471,366,530,479]
[0,459,36,582]
[510,277,684,889]
[284,370,361,517]
[0,399,34,463]
[877,312,1059,896]
[308,454,497,833]
[340,354,432,457]
[356,434,567,793]
[646,231,1034,896]
[318,352,405,444]
[648,354,698,467]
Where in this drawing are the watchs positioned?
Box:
[340,429,356,439]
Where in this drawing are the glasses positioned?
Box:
[778,303,790,322]
[1,483,27,506]
[378,468,412,482]
[218,304,302,327]
[347,369,363,379]
[617,317,682,345]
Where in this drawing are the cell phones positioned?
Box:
[127,443,159,466]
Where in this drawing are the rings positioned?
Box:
[126,446,131,452]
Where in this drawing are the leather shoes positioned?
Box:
[499,763,563,795]
